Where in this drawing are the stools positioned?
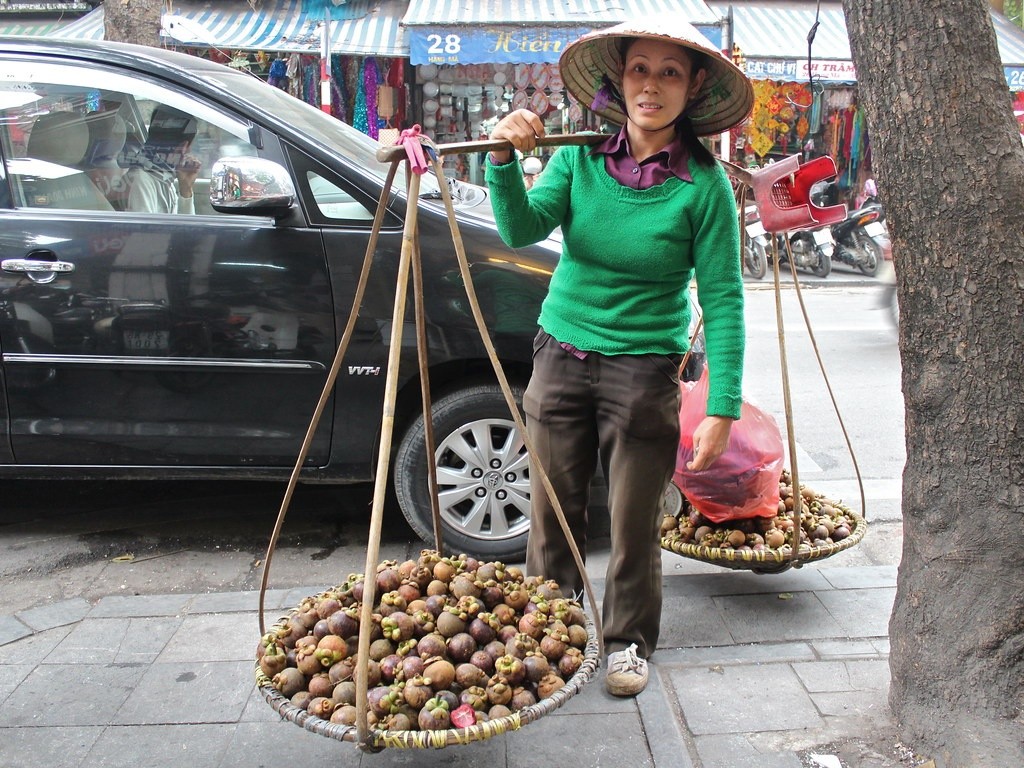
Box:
[750,153,848,233]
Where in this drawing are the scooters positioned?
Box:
[732,203,771,277]
[810,179,893,277]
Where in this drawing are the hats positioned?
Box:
[558,13,755,137]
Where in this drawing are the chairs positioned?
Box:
[24,111,115,211]
[82,111,127,210]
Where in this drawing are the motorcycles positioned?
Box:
[766,224,836,277]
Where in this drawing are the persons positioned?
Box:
[485,12,756,696]
[116,104,201,215]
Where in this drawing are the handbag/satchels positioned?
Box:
[671,365,785,524]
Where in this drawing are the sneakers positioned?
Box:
[606,643,648,694]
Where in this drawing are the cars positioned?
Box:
[2,38,709,563]
